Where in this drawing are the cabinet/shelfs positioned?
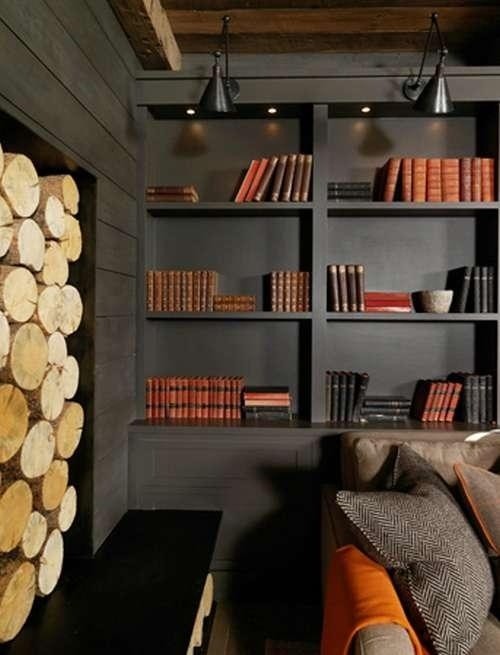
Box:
[127,66,500,598]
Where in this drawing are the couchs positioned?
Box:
[318,427,500,655]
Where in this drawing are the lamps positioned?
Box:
[402,11,456,114]
[197,15,241,114]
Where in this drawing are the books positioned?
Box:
[144,185,199,203]
[327,264,412,313]
[446,266,497,313]
[144,375,293,424]
[380,156,495,203]
[325,370,411,424]
[408,371,496,425]
[271,271,311,312]
[327,181,372,202]
[235,153,312,202]
[146,269,257,312]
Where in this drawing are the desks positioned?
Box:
[0,506,223,654]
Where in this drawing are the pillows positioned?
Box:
[334,441,494,655]
[353,430,500,492]
[452,460,500,559]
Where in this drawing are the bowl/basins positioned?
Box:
[412,289,453,313]
